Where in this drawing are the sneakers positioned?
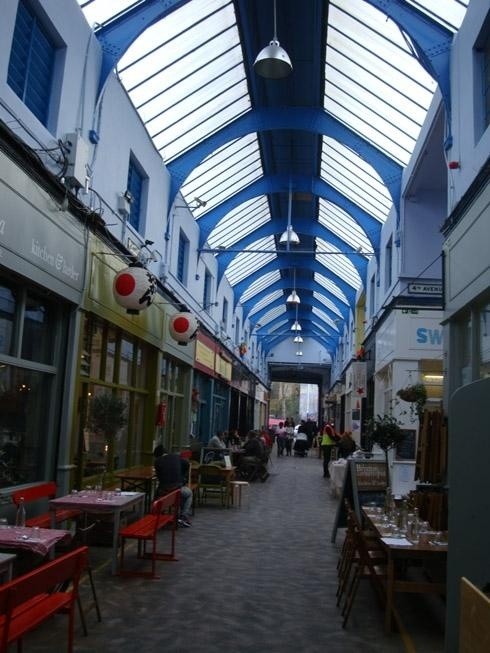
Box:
[324,473,330,477]
[178,516,190,527]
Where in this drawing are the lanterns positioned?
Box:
[112,262,159,315]
[168,308,200,346]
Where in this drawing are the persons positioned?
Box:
[321,416,342,479]
[205,426,275,484]
[153,444,194,527]
[273,415,325,459]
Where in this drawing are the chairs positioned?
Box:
[333,498,389,629]
[198,465,225,505]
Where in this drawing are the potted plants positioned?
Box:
[395,370,425,405]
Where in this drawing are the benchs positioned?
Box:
[229,479,249,506]
[0,481,182,649]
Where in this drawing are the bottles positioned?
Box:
[14,494,26,536]
[384,493,421,540]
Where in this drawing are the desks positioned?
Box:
[116,464,157,512]
[189,463,237,508]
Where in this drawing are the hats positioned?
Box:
[154,445,168,456]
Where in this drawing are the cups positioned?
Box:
[71,481,122,500]
[0,517,7,533]
[31,525,39,537]
[365,489,438,545]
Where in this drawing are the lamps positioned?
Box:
[252,3,293,77]
